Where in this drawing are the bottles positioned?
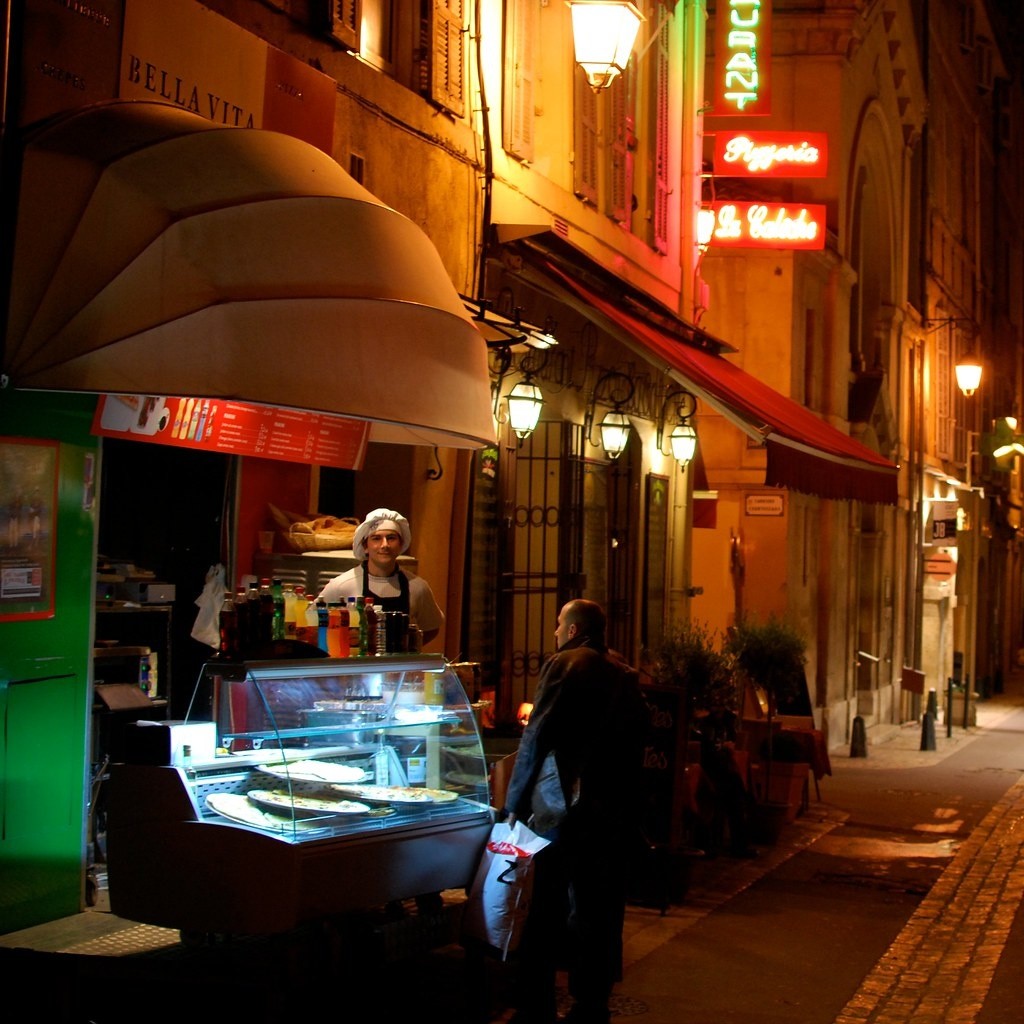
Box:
[171,397,218,443]
[216,576,386,658]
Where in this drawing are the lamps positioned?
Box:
[494,347,550,447]
[583,371,635,465]
[568,0,647,94]
[921,316,985,400]
[657,390,698,473]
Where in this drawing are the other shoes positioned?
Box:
[559,1002,611,1024]
[505,1009,557,1024]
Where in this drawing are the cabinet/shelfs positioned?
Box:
[94,601,174,763]
[175,654,491,845]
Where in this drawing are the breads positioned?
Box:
[267,502,358,535]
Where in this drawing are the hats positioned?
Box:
[353,509,411,560]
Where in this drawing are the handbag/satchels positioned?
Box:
[467,817,551,961]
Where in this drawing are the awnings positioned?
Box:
[0,98,500,449]
[507,254,900,509]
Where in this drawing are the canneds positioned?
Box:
[424,661,482,705]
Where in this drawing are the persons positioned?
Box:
[312,509,444,652]
[504,598,649,1024]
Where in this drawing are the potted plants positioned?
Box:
[643,615,736,884]
[721,615,816,840]
